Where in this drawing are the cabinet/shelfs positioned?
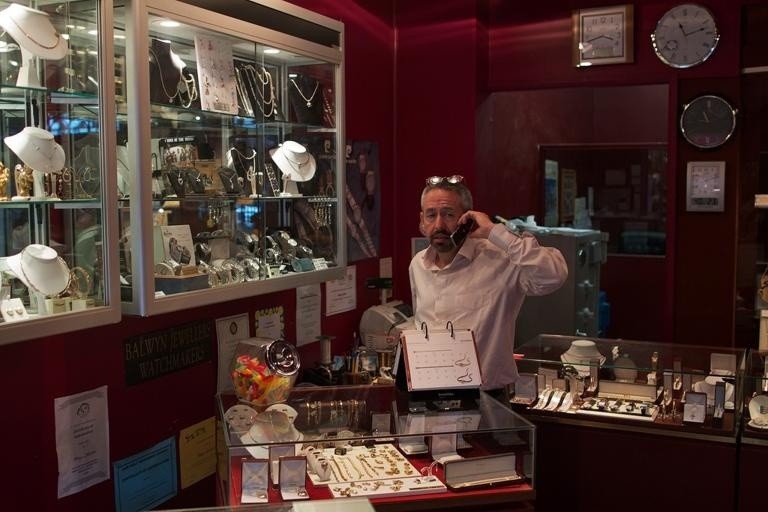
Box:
[59,0,348,319]
[0,0,122,346]
[213,387,537,512]
[514,335,768,512]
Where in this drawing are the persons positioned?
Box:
[395,175,570,455]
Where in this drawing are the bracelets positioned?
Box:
[660,397,678,422]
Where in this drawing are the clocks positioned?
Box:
[686,161,726,212]
[649,0,722,70]
[678,94,740,152]
[572,4,634,69]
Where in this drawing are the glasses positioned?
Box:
[425,175,466,188]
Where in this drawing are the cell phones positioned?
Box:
[449,218,474,248]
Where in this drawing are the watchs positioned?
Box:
[583,395,655,417]
[194,224,315,289]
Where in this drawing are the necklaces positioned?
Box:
[172,169,185,187]
[31,141,60,170]
[177,72,197,110]
[189,169,203,182]
[242,63,275,118]
[230,146,257,177]
[148,46,182,104]
[280,144,311,166]
[291,78,320,107]
[3,10,60,49]
[117,157,130,173]
[19,246,72,298]
[246,427,301,450]
[281,460,308,497]
[242,458,267,501]
